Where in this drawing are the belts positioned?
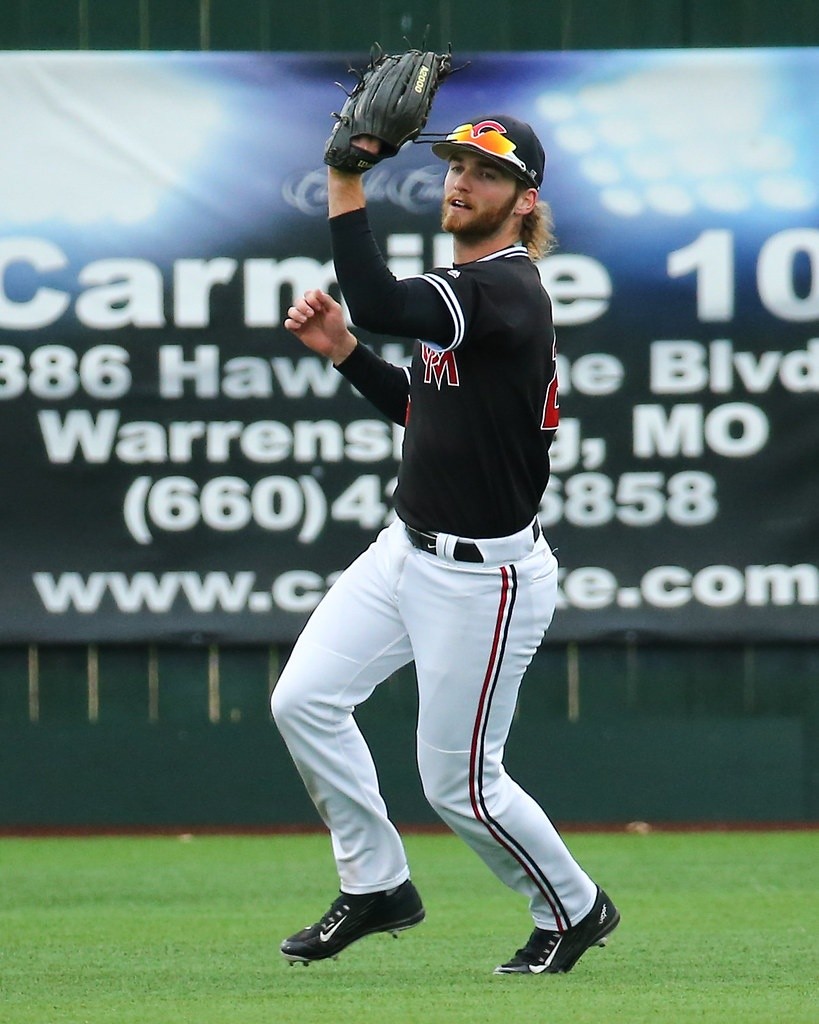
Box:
[406,516,540,563]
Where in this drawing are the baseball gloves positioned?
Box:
[323,50,449,175]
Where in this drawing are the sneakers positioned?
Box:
[280,878,425,966]
[493,884,620,974]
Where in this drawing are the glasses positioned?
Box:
[446,124,526,172]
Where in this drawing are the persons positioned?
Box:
[274,39,622,983]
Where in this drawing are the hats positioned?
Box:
[431,114,545,190]
